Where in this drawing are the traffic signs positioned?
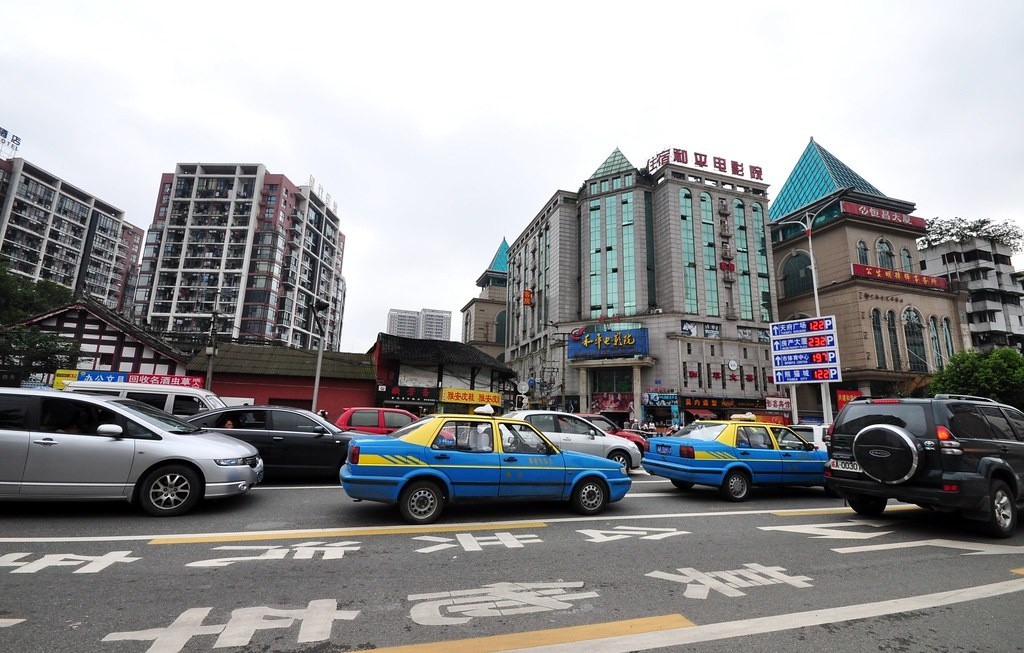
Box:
[772,364,843,384]
[770,331,839,354]
[769,315,837,337]
[772,347,840,369]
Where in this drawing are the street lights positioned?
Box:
[766,185,857,429]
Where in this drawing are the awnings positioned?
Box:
[687,409,717,419]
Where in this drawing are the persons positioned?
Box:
[669,422,678,434]
[484,428,516,452]
[185,400,199,413]
[680,424,684,430]
[592,393,634,413]
[243,403,255,423]
[641,393,659,406]
[318,410,327,419]
[694,414,699,421]
[643,420,655,438]
[223,418,235,429]
[624,419,641,434]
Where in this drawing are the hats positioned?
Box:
[634,419,638,423]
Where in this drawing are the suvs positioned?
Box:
[824,392,1024,539]
[776,422,831,455]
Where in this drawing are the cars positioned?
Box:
[182,404,378,483]
[339,402,632,525]
[0,387,265,518]
[466,408,642,476]
[639,412,827,503]
[541,413,648,460]
[335,406,455,447]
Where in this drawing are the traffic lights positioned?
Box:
[516,395,524,409]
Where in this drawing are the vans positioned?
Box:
[60,379,228,433]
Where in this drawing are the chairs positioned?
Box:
[41,411,56,432]
[84,405,100,432]
[469,430,492,452]
[749,434,768,449]
